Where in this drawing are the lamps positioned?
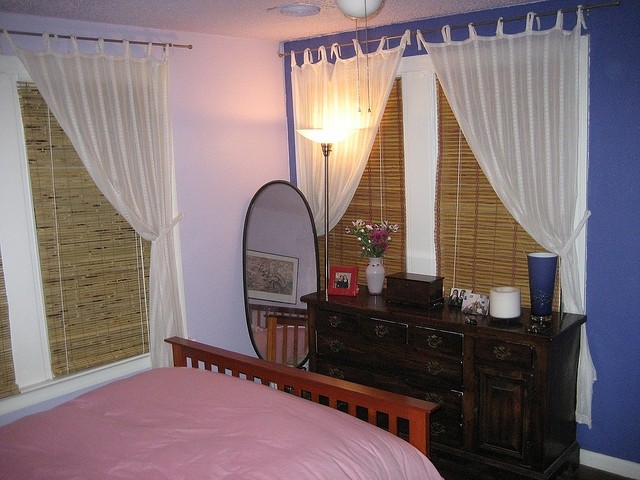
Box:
[334,0,385,112]
[296,123,354,296]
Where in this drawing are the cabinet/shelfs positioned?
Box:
[296,282,589,480]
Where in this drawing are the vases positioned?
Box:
[365,258,385,294]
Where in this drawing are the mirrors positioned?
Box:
[243,180,318,368]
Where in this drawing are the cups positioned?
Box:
[527,252,558,323]
[490,285,521,318]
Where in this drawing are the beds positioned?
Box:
[0,336,446,479]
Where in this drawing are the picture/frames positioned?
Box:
[328,265,357,296]
[246,249,298,305]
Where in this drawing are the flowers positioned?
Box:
[345,219,399,258]
